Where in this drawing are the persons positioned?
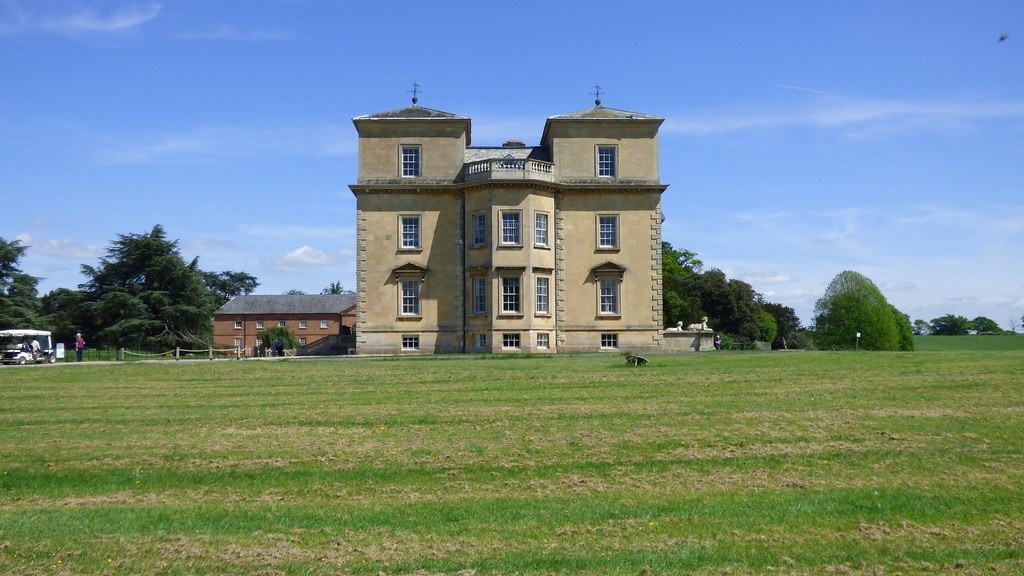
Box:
[31,337,43,354]
[271,337,284,357]
[715,335,721,351]
[75,333,85,362]
[21,337,33,352]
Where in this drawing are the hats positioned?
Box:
[75,333,82,337]
